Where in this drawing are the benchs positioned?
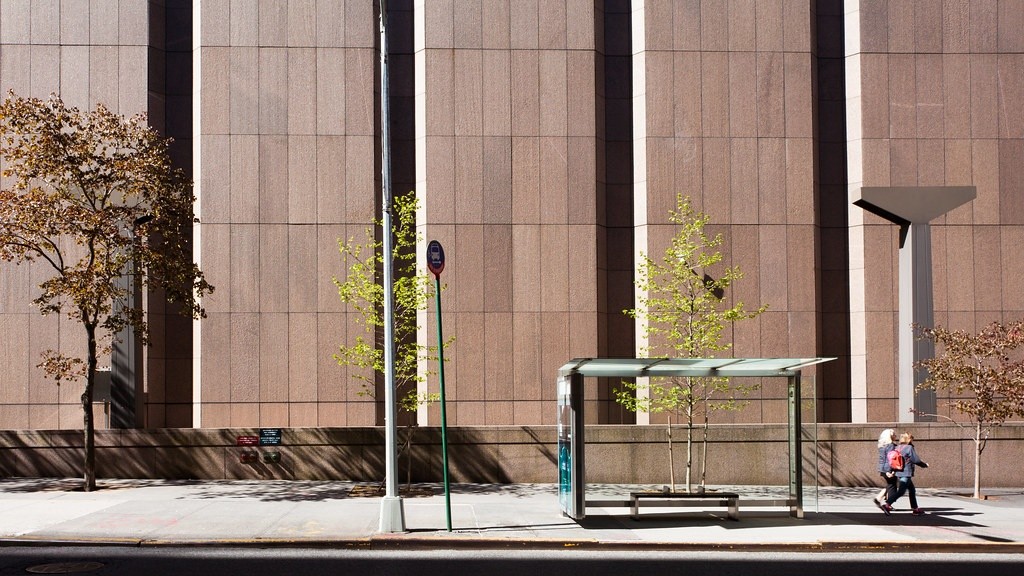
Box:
[629,492,740,522]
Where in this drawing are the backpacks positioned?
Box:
[887,444,910,470]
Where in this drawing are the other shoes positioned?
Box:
[874,498,893,509]
[881,506,892,516]
[912,508,925,516]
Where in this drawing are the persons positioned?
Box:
[873,428,929,516]
